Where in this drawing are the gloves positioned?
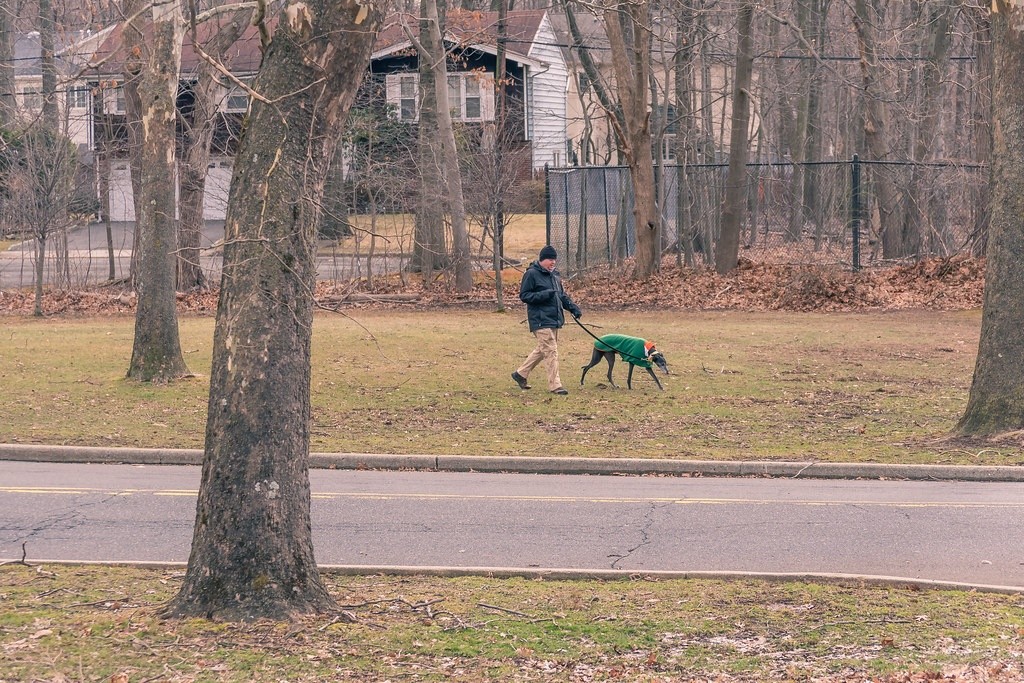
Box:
[574,314,582,319]
[549,290,558,298]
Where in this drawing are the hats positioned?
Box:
[540,245,557,261]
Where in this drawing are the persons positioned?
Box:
[511,245,583,395]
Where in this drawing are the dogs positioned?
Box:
[579,333,670,392]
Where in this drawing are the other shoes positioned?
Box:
[512,372,531,389]
[553,387,568,395]
